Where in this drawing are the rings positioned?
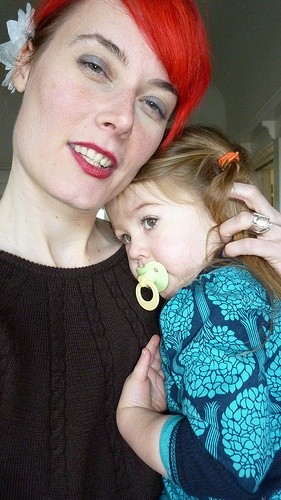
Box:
[247,212,272,235]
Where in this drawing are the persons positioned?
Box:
[105,124,281,500]
[0,0,281,500]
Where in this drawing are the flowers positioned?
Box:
[0,2,35,94]
